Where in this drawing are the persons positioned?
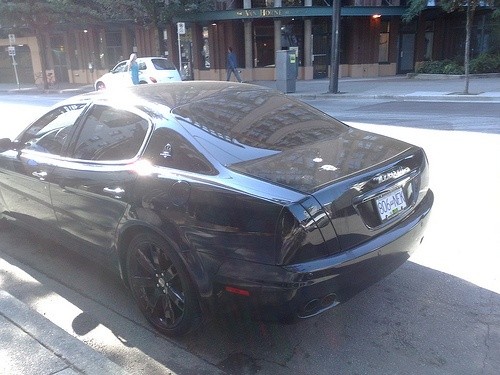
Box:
[128,53,140,85]
[226,47,243,83]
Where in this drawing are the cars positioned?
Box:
[94,57,183,92]
[1,80,435,338]
